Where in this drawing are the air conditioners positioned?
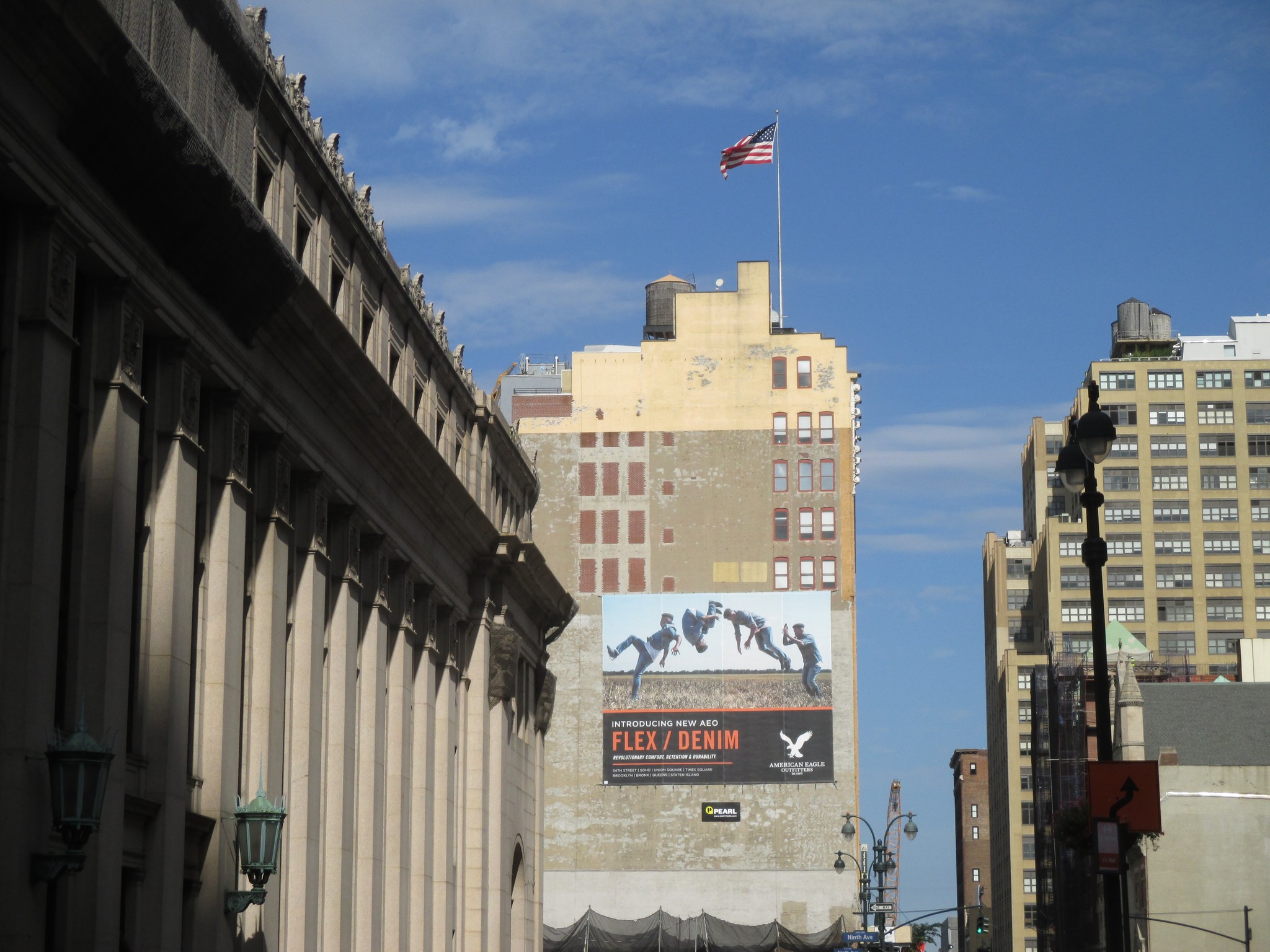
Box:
[1053,513,1071,523]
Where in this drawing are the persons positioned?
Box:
[781,622,826,707]
[723,606,791,672]
[607,612,681,700]
[682,601,724,653]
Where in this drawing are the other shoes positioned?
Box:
[820,695,824,701]
[779,659,785,670]
[783,656,791,672]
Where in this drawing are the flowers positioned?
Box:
[1050,798,1092,847]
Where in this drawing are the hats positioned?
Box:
[697,645,708,653]
[792,623,805,628]
[661,613,674,620]
[723,608,732,619]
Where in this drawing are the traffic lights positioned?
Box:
[982,917,989,934]
[977,915,983,934]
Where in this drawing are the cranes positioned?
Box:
[882,777,902,928]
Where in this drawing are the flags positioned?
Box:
[718,118,777,181]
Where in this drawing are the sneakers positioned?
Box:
[709,600,723,608]
[606,645,617,660]
[715,608,722,615]
[630,695,638,701]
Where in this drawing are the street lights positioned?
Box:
[1054,380,1133,951]
[840,811,919,952]
[833,850,896,952]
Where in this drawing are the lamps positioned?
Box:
[30,684,116,883]
[223,751,287,916]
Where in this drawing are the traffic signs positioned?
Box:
[868,903,894,913]
